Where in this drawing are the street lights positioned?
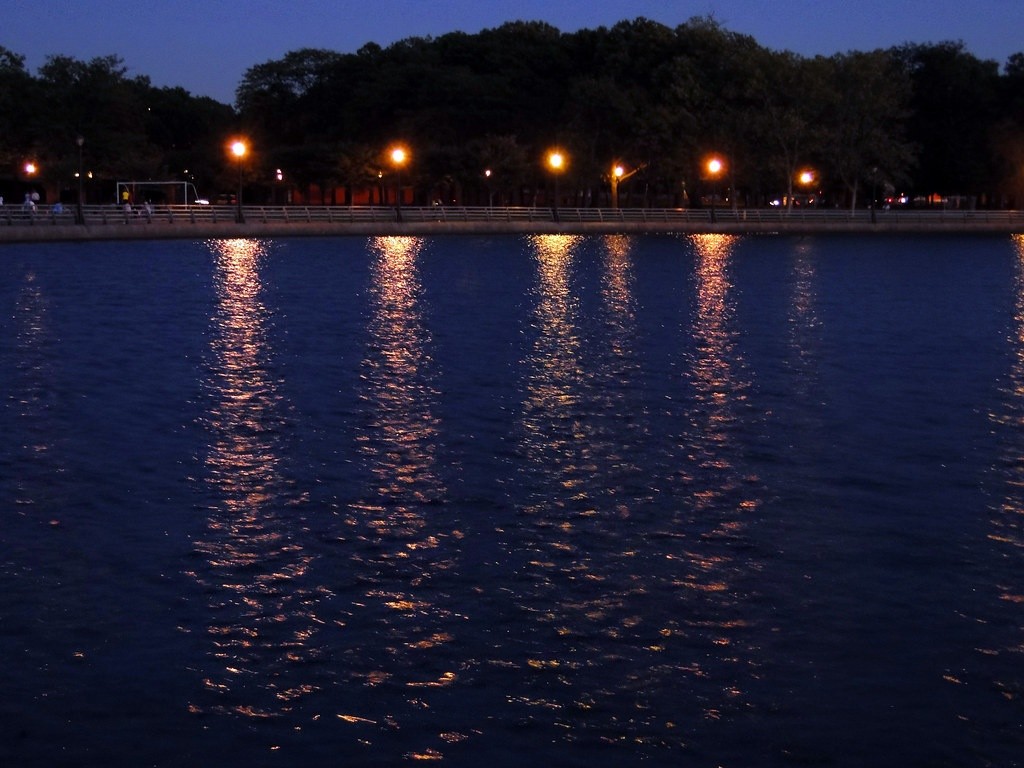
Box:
[394,149,405,222]
[615,167,623,207]
[550,155,562,223]
[231,141,246,223]
[800,173,811,207]
[76,135,85,224]
[708,160,720,223]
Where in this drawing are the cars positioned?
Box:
[881,191,927,209]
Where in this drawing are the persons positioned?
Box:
[22,196,42,220]
[123,189,128,205]
[0,200,12,226]
